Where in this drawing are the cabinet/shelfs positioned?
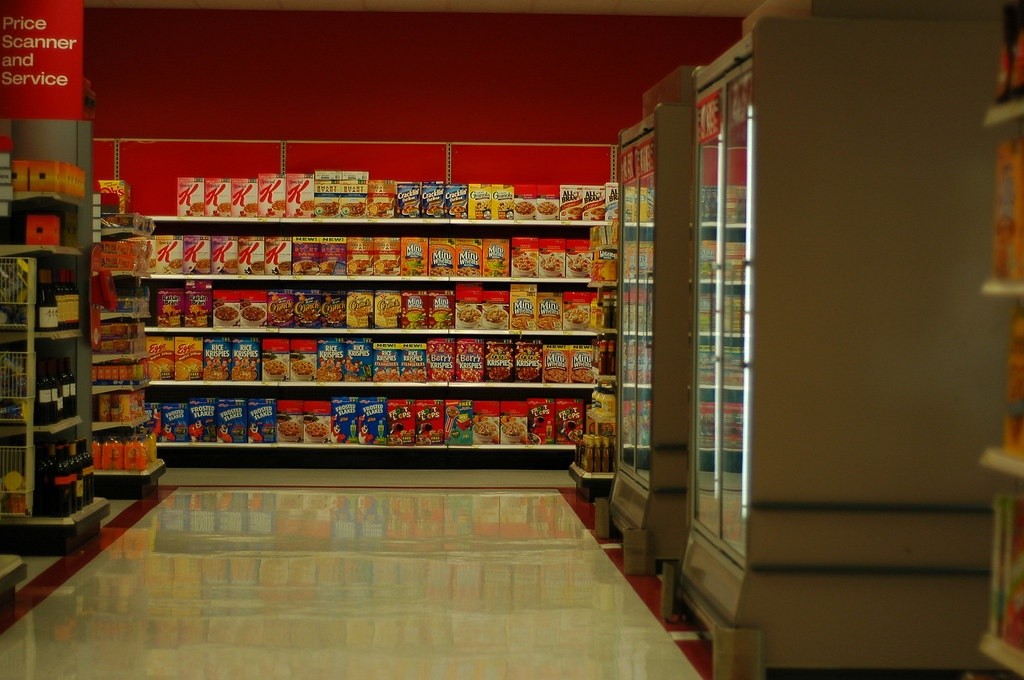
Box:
[92,225,168,500]
[700,219,743,455]
[623,218,652,452]
[567,245,618,501]
[977,98,1024,680]
[143,214,614,470]
[0,192,111,559]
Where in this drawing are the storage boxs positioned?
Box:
[10,161,86,198]
[26,213,61,246]
[702,184,742,452]
[91,170,618,444]
[622,185,653,449]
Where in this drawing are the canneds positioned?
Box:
[90,361,144,382]
